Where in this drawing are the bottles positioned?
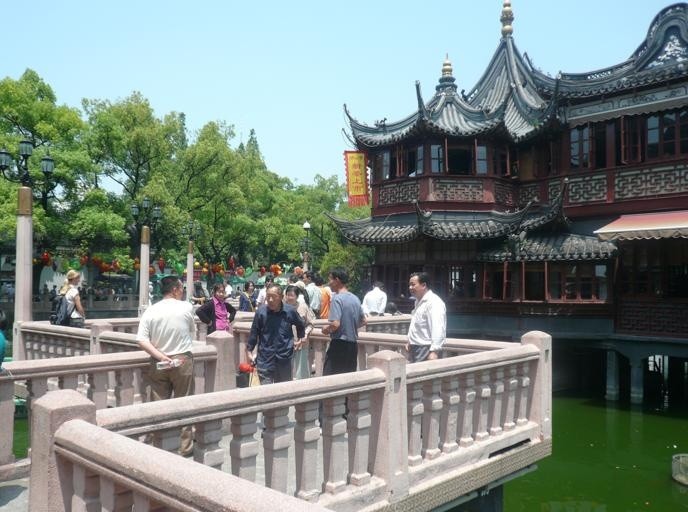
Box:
[155,359,186,370]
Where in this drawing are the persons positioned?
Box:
[387,302,402,316]
[236,281,305,441]
[137,276,200,458]
[363,282,387,317]
[151,278,161,299]
[407,270,447,440]
[318,267,368,433]
[60,270,85,328]
[78,283,133,301]
[285,286,317,381]
[223,279,233,300]
[196,283,236,335]
[192,279,210,304]
[239,269,333,320]
[42,284,57,302]
[0,310,9,370]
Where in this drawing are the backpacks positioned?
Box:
[48,287,76,326]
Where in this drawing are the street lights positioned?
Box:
[302,221,311,273]
[181,218,202,304]
[0,135,55,320]
[131,196,162,318]
